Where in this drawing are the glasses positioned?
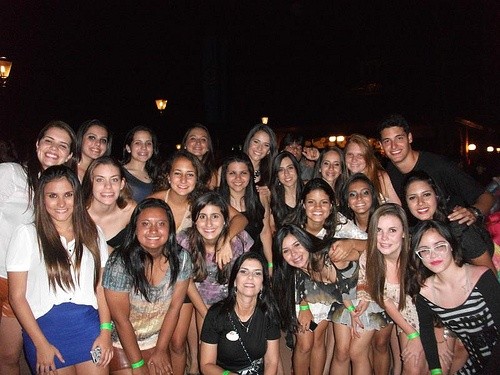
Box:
[346,153,366,159]
[415,242,449,259]
[287,144,302,150]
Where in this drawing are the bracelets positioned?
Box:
[100,322,113,329]
[266,262,273,268]
[431,368,442,375]
[408,331,420,339]
[470,207,481,216]
[223,370,230,375]
[300,304,309,310]
[348,305,354,311]
[131,359,145,369]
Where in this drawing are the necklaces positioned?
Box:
[238,312,256,332]
[144,176,147,179]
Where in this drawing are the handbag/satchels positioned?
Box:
[237,358,264,375]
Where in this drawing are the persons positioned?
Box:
[0,122,500,375]
